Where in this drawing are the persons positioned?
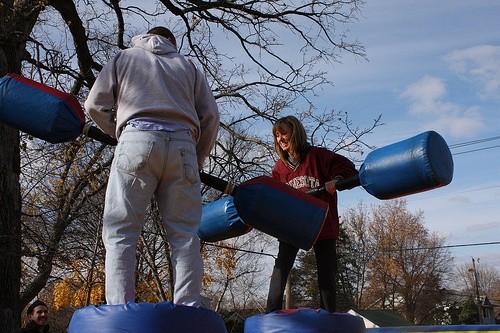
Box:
[265,116,356,314]
[19,301,50,333]
[83,27,220,307]
